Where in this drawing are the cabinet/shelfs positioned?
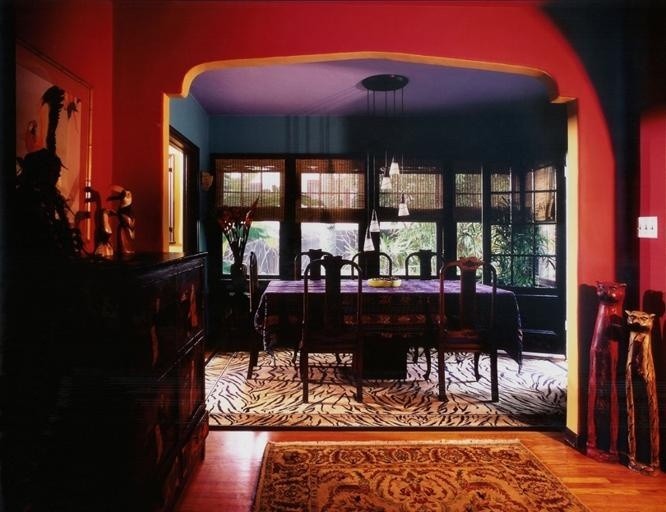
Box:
[0,251,210,512]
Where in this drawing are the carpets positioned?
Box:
[203,348,567,433]
[251,439,591,512]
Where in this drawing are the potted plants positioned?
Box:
[205,195,260,338]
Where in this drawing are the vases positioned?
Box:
[625,309,660,472]
[586,281,626,464]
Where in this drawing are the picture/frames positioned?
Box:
[16,33,94,246]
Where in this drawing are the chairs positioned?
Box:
[246,249,499,404]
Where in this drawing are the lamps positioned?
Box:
[361,74,409,252]
